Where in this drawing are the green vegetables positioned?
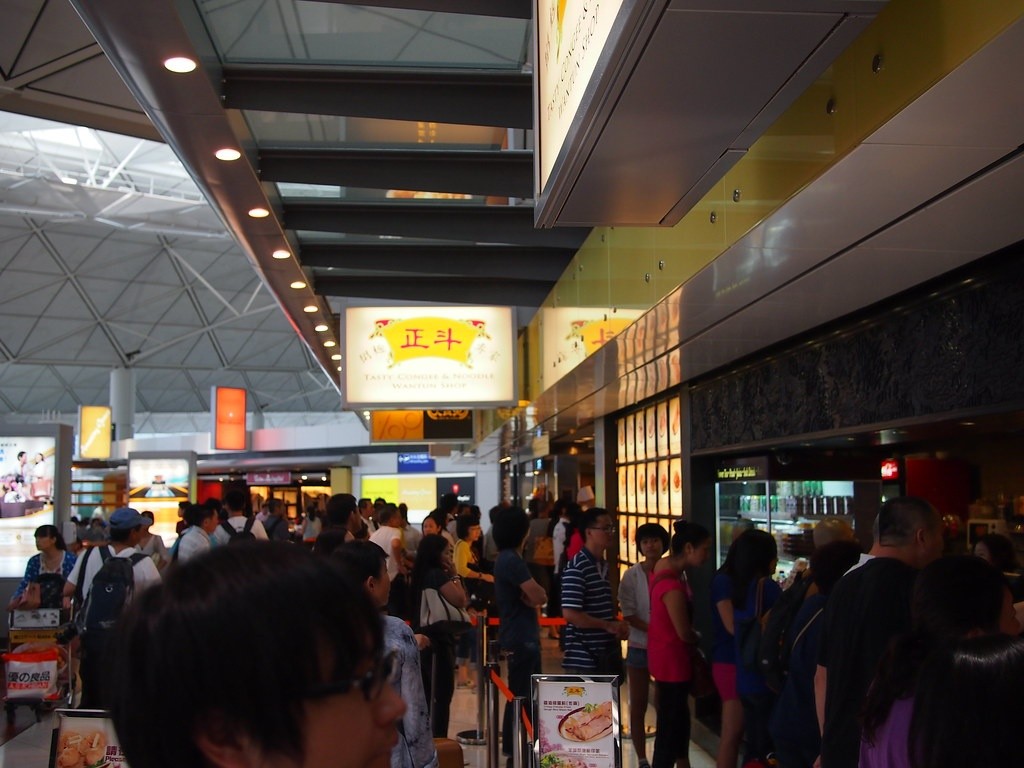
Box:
[540,753,563,768]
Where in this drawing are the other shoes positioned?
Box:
[639,760,650,768]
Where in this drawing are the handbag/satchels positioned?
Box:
[418,568,472,635]
[593,643,627,686]
[690,646,714,697]
[8,582,42,609]
[758,577,814,695]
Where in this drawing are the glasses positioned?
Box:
[308,647,399,702]
[590,524,616,533]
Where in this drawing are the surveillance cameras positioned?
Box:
[777,452,793,465]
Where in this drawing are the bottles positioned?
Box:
[720,520,817,592]
[941,485,1024,543]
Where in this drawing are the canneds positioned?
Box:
[739,494,852,518]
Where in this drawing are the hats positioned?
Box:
[109,509,153,529]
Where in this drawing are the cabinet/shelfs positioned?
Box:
[714,479,880,591]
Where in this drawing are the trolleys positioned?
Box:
[3,596,76,722]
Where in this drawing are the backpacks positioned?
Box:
[76,545,149,646]
[221,515,257,546]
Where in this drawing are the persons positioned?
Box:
[711,517,881,768]
[335,540,440,768]
[7,508,167,714]
[170,491,291,568]
[560,507,713,768]
[297,494,586,768]
[101,539,407,767]
[907,633,1024,768]
[857,555,1024,768]
[813,497,944,768]
[973,534,1018,572]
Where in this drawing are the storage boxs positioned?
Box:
[13,606,71,628]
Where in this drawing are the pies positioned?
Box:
[564,701,611,740]
